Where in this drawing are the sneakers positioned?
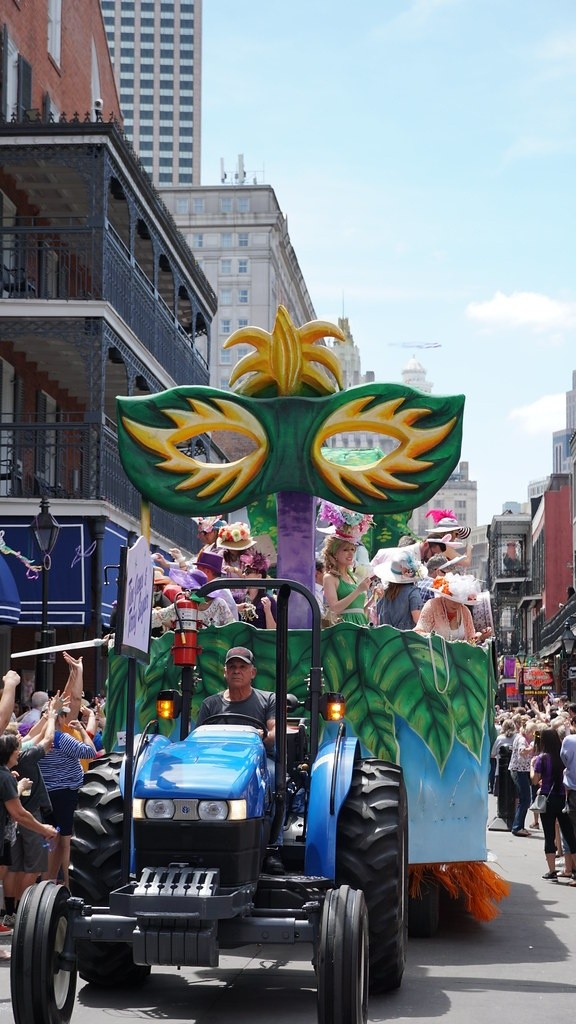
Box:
[0,923,13,935]
[3,913,17,926]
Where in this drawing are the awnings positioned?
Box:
[0,554,21,624]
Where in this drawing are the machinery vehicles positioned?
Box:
[9,576,408,1024]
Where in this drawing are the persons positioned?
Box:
[0,650,106,936]
[103,519,277,645]
[195,646,285,872]
[491,692,576,887]
[314,517,492,646]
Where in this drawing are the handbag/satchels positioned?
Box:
[529,795,547,813]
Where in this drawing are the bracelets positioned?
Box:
[43,716,47,720]
[180,565,186,568]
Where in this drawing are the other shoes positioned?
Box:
[568,879,576,886]
[541,872,557,879]
[557,872,572,877]
[513,829,531,836]
[530,823,539,829]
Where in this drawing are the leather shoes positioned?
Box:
[262,850,285,873]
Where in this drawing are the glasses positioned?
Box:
[242,568,259,574]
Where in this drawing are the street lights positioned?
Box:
[30,488,61,690]
[517,642,527,707]
[559,621,576,702]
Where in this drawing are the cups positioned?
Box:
[22,781,33,796]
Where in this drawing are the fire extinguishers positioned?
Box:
[174,587,201,667]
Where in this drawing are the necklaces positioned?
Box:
[442,599,461,643]
[428,634,450,693]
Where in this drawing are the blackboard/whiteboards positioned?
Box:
[122,535,154,654]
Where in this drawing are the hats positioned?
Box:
[192,552,227,575]
[169,569,220,598]
[217,522,257,551]
[428,573,489,605]
[316,502,373,545]
[373,551,428,584]
[42,701,70,713]
[425,510,471,539]
[225,647,253,665]
[239,550,270,571]
[154,571,170,584]
